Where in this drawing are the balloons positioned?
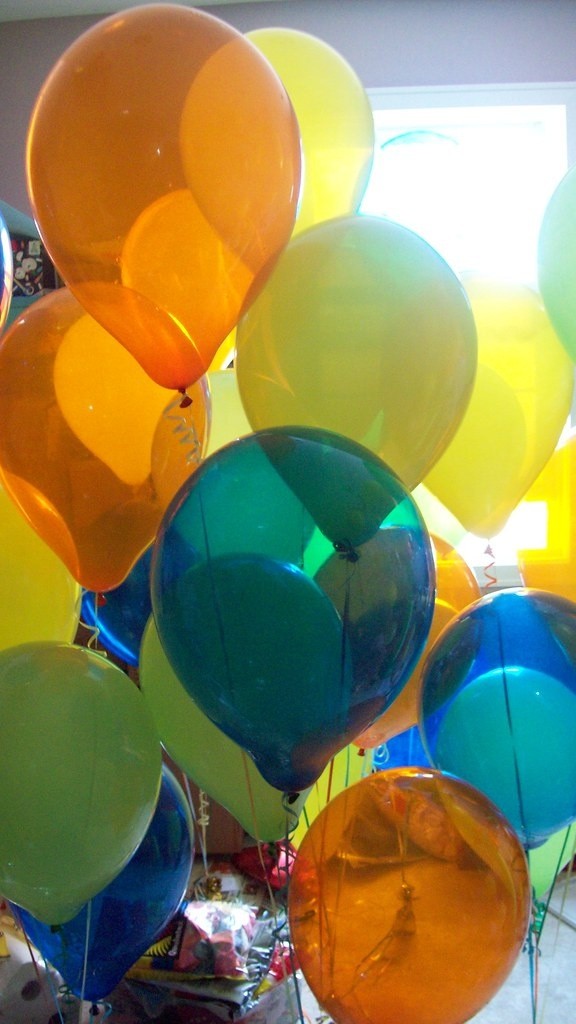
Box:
[9,763,195,1016]
[0,641,163,934]
[0,3,576,854]
[288,766,531,1024]
